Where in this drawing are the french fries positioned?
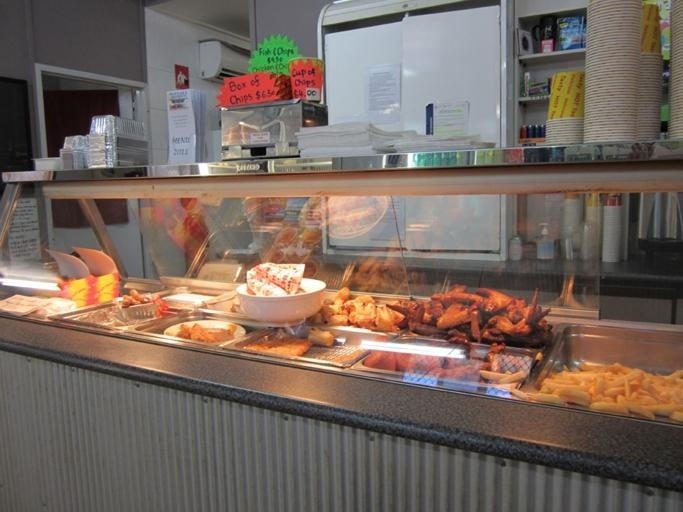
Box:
[479,361,683,425]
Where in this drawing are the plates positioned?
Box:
[163,319,247,345]
[316,193,389,240]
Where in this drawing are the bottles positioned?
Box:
[508,233,522,261]
[521,123,545,138]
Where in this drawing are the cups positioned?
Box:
[545,70,585,148]
[561,191,623,264]
[532,13,557,51]
[288,57,325,104]
[636,4,663,142]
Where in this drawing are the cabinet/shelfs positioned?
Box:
[0,141,683,512]
[517,6,585,101]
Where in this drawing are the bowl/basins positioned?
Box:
[235,278,327,322]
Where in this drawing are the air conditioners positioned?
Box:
[199,40,251,81]
